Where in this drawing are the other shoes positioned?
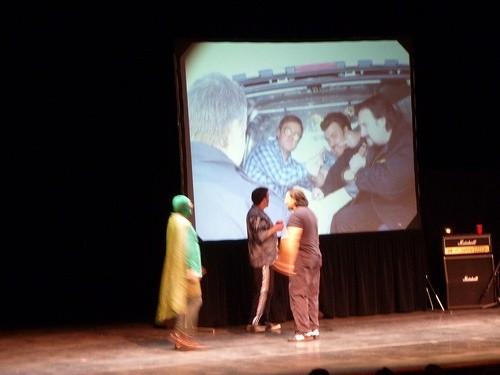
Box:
[288,334,305,341]
[304,329,320,339]
[247,324,266,332]
[264,322,281,330]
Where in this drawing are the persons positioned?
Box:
[187,74,293,239]
[320,113,362,192]
[247,188,284,334]
[331,94,416,229]
[283,194,321,342]
[246,116,310,194]
[376,368,394,375]
[309,367,330,375]
[424,363,446,374]
[156,194,214,350]
[408,371,419,374]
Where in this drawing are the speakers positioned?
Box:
[438,254,496,310]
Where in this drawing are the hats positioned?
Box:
[172,195,194,218]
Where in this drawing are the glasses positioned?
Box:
[282,127,301,142]
